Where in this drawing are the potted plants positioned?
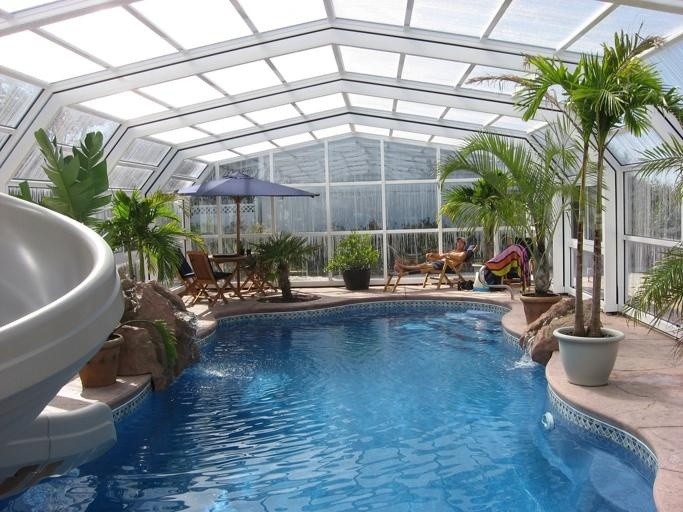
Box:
[430,115,613,326]
[320,229,382,291]
[19,129,123,391]
[462,21,667,387]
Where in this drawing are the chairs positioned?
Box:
[172,246,278,306]
[384,242,479,293]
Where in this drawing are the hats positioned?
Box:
[456,236,466,245]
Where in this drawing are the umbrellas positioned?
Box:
[174,171,320,293]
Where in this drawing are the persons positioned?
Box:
[394,237,467,276]
